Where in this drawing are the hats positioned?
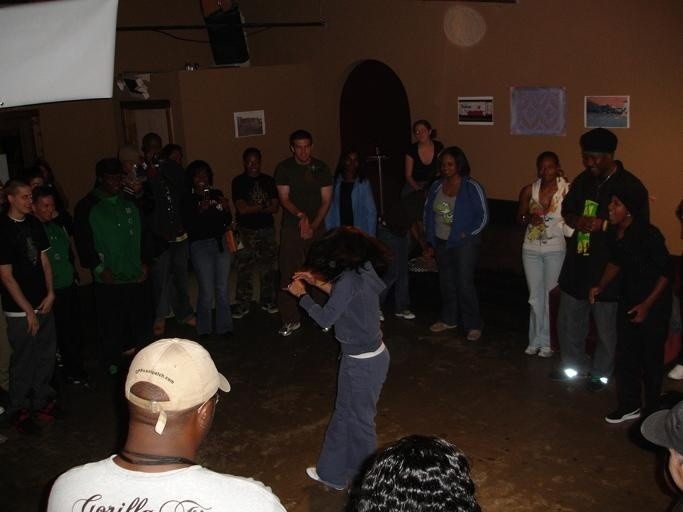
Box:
[578,127,619,154]
[124,337,232,415]
[96,157,129,177]
[639,399,683,457]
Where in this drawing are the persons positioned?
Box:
[347,432,482,512]
[515,127,682,423]
[282,225,390,495]
[641,401,681,512]
[2,159,93,437]
[46,336,287,512]
[74,132,236,354]
[231,117,491,341]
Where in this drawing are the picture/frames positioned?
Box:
[456,95,497,126]
[583,94,632,130]
[233,110,266,138]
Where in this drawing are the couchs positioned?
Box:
[401,198,528,335]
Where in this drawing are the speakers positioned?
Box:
[205,7,249,65]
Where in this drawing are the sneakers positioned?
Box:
[379,306,416,323]
[429,320,457,333]
[13,410,58,433]
[467,321,485,341]
[261,301,280,314]
[278,319,301,338]
[231,304,251,320]
[525,344,554,359]
[586,377,607,392]
[666,362,682,381]
[304,466,349,491]
[550,370,587,381]
[603,405,641,425]
